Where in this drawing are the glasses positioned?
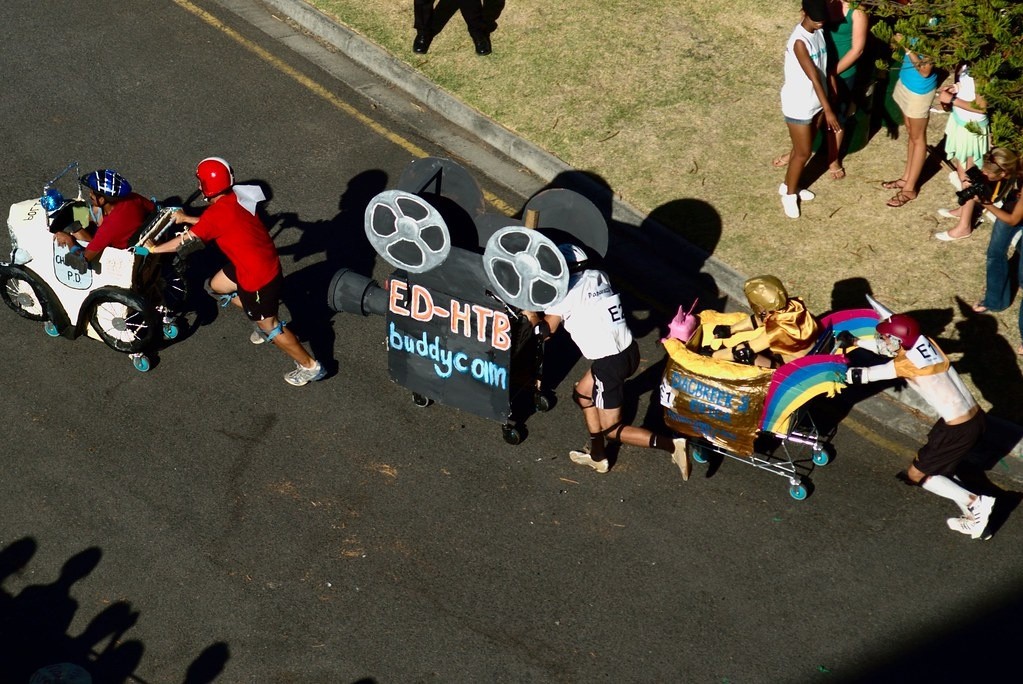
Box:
[987,148,1006,172]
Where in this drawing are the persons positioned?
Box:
[713,275,819,369]
[836,315,996,539]
[144,157,327,387]
[524,244,688,480]
[772,0,1023,354]
[52,170,156,262]
[413,0,491,55]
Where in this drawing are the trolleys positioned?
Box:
[325,157,610,447]
[0,158,189,373]
[659,334,859,501]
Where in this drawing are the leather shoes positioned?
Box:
[414,29,430,52]
[473,34,492,55]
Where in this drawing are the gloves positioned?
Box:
[836,331,856,348]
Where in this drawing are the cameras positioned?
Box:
[956,178,988,206]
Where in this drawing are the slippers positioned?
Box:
[936,230,971,241]
[938,209,960,219]
[972,299,989,313]
[774,154,790,168]
[829,167,844,181]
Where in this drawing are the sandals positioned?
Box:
[881,179,907,190]
[887,189,916,207]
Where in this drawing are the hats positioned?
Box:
[802,0,829,22]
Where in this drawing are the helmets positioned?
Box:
[743,275,787,312]
[194,156,235,198]
[864,292,920,348]
[559,243,589,270]
[81,169,132,197]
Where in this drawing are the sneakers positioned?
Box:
[569,451,609,473]
[672,437,689,481]
[778,182,815,200]
[947,516,994,540]
[250,330,268,345]
[781,191,800,219]
[970,495,996,539]
[285,360,327,386]
